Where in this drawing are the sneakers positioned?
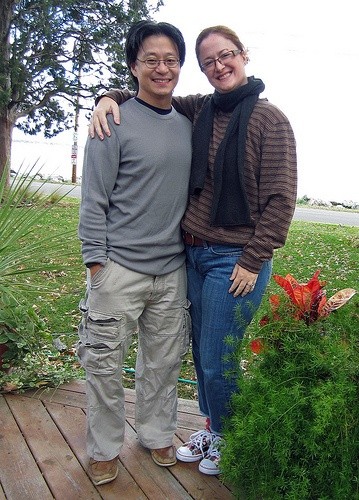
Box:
[198,437,225,475]
[176,428,220,462]
[149,443,177,466]
[88,457,119,486]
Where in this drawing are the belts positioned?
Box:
[183,232,212,247]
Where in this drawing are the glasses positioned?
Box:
[136,57,181,69]
[199,49,242,72]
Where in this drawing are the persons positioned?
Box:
[89,26,298,474]
[79,21,194,484]
